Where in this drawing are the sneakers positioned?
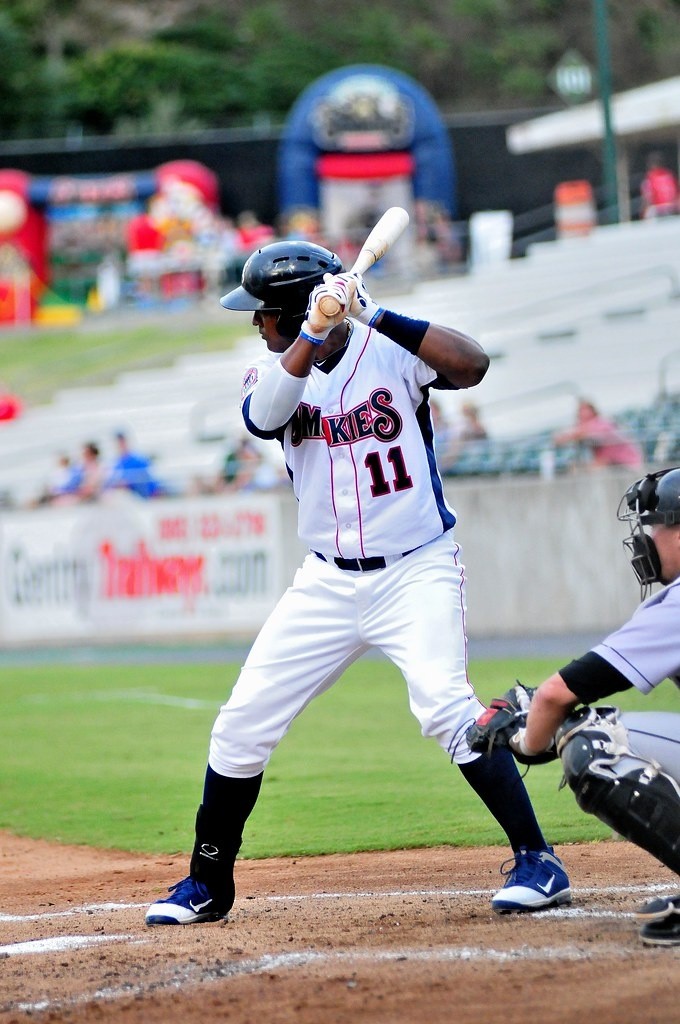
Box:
[491,845,572,915]
[145,876,234,924]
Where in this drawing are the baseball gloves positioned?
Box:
[463,683,559,765]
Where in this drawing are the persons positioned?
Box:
[23,430,171,511]
[554,400,642,473]
[189,439,295,492]
[145,238,574,931]
[453,467,680,947]
[637,148,680,222]
[424,399,488,474]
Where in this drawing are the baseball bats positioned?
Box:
[318,206,410,314]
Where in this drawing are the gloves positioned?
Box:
[505,714,559,765]
[323,271,384,327]
[300,279,357,346]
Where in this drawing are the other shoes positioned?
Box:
[639,911,680,949]
[637,897,680,921]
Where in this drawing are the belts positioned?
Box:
[313,545,421,571]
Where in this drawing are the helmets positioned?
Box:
[220,241,345,339]
[638,466,680,525]
